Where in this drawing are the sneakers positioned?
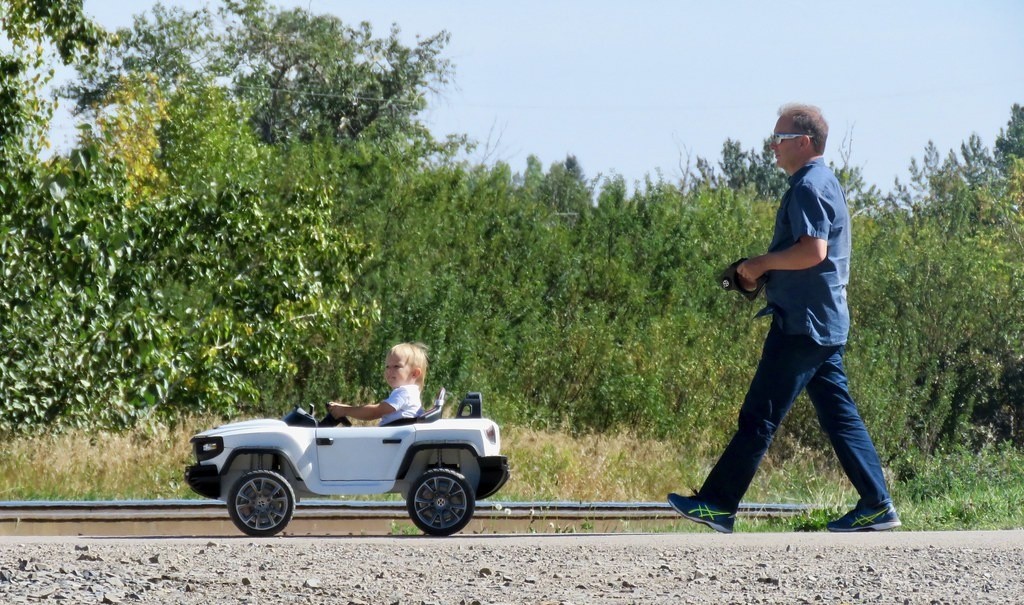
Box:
[667,489,735,533]
[826,499,902,532]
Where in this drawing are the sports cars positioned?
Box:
[183,389,511,536]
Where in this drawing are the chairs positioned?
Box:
[416,387,445,424]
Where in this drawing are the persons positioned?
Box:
[327,343,429,426]
[667,106,901,532]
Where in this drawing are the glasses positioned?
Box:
[774,132,816,144]
